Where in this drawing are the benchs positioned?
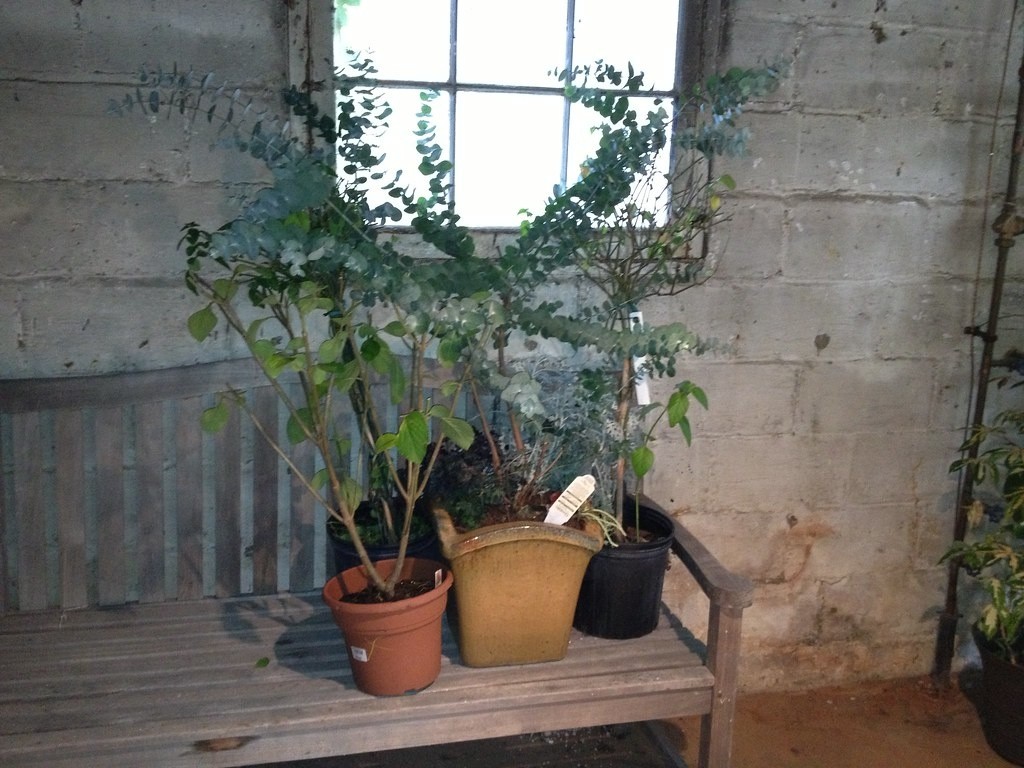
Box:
[0,349,755,767]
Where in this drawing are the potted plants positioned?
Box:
[938,347,1024,766]
[132,38,794,695]
